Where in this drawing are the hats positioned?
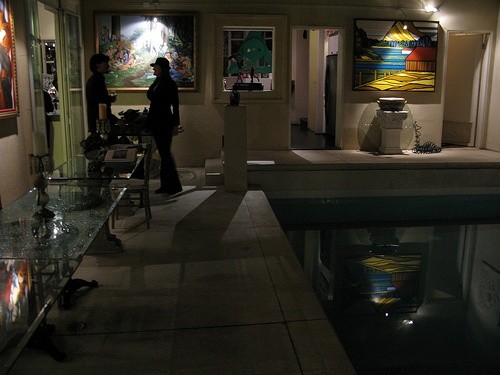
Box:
[150,57,171,69]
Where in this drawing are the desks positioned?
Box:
[0,181,128,375]
[45,125,153,230]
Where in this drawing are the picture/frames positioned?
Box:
[352,17,440,93]
[94,9,200,92]
[0,0,20,119]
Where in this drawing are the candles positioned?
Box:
[98,102,108,120]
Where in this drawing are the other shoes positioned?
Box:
[167,187,184,199]
[155,185,166,194]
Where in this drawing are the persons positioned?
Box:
[146,58,183,196]
[43,89,54,149]
[86,53,117,132]
